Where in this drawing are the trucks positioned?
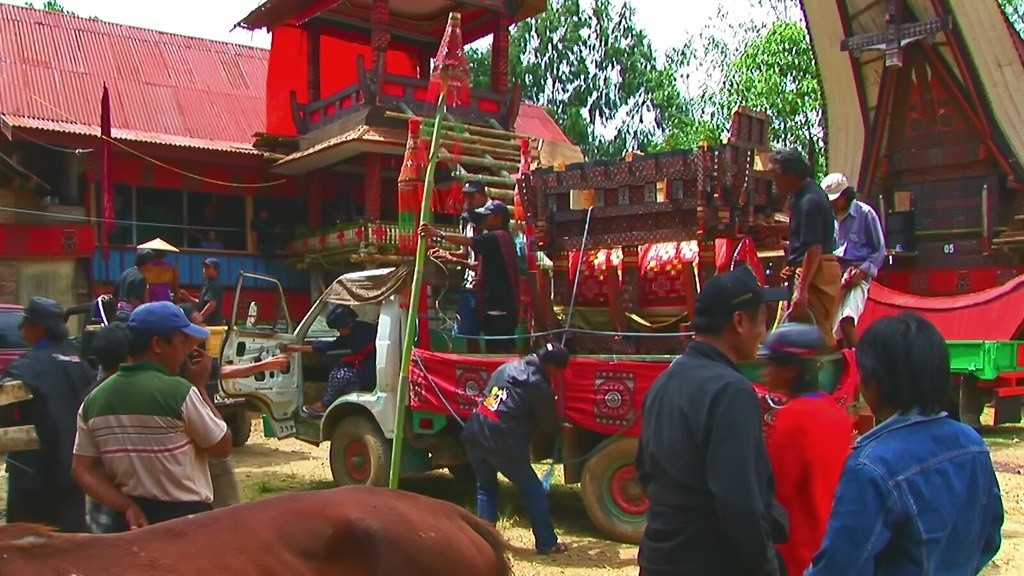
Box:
[216,266,868,544]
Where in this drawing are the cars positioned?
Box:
[0,303,33,425]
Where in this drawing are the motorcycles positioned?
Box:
[62,301,251,448]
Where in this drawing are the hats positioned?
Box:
[545,341,569,367]
[128,300,209,340]
[476,200,509,216]
[820,172,848,200]
[461,180,485,192]
[201,258,220,269]
[693,264,792,312]
[326,304,357,328]
[761,322,827,365]
[136,237,180,253]
[13,297,63,323]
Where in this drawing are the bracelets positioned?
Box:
[438,230,445,239]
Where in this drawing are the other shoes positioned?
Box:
[537,543,568,555]
[303,405,325,416]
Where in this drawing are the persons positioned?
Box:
[279,304,379,416]
[428,179,490,354]
[637,267,795,575]
[767,151,842,349]
[202,230,227,250]
[71,301,232,533]
[255,206,282,251]
[756,324,856,576]
[820,172,886,349]
[801,312,1004,575]
[0,297,98,531]
[179,257,223,326]
[458,344,575,555]
[111,248,155,323]
[135,238,179,303]
[94,324,132,384]
[417,200,523,356]
